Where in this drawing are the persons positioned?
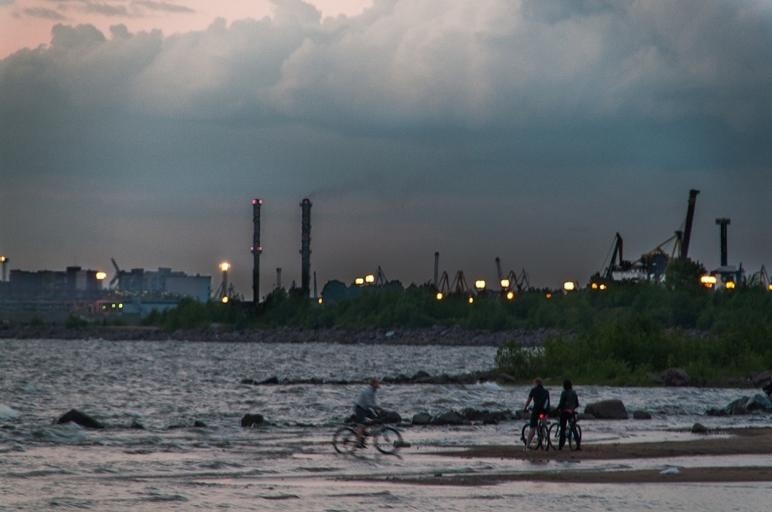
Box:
[350,378,387,448]
[523,376,550,450]
[554,378,584,451]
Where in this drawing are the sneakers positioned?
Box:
[355,437,368,449]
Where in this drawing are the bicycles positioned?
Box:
[331,408,405,457]
[548,408,584,451]
[518,408,552,451]
